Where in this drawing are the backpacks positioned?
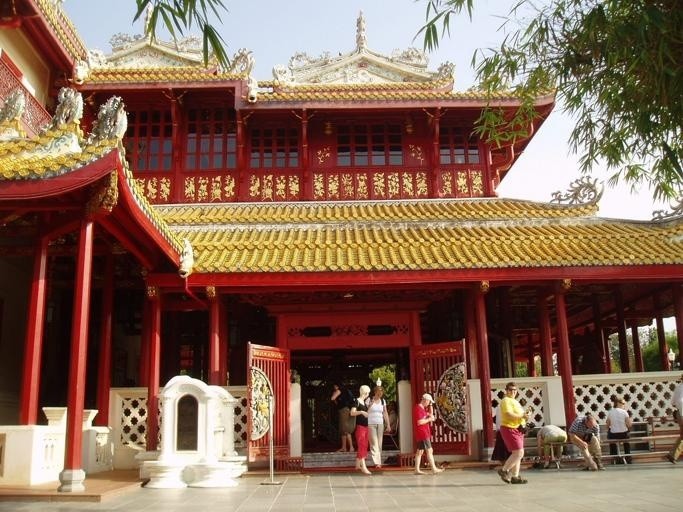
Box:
[346,410,358,434]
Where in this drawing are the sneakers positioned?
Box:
[665,455,678,466]
[510,476,529,485]
[498,468,511,484]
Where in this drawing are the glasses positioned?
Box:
[506,388,517,392]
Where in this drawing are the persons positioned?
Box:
[413,394,445,475]
[568,415,605,471]
[491,382,532,484]
[537,424,567,468]
[664,375,683,464]
[331,381,391,475]
[607,398,633,464]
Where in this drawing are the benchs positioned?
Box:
[542,431,643,469]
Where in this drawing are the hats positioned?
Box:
[421,393,436,404]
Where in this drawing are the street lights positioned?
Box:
[667,348,675,371]
